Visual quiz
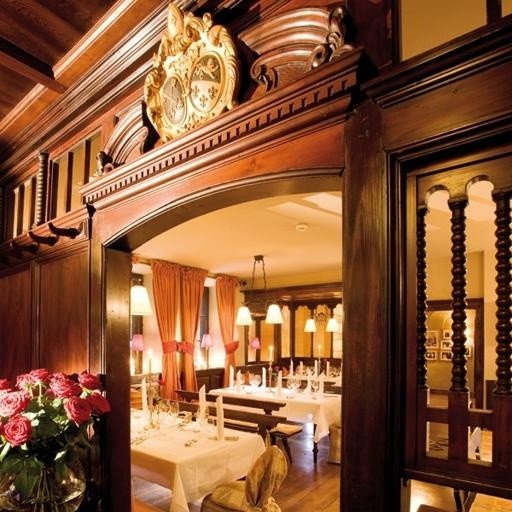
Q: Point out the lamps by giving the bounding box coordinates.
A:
[235,255,283,325]
[325,302,339,333]
[131,334,144,374]
[250,337,261,350]
[130,278,153,339]
[303,303,317,333]
[201,334,214,369]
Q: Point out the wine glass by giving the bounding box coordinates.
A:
[152,399,179,430]
[288,378,301,399]
[235,374,261,395]
[310,380,319,400]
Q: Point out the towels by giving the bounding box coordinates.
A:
[229,359,330,393]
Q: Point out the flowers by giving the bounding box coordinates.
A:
[0,368,112,502]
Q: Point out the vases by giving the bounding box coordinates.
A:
[0,443,87,512]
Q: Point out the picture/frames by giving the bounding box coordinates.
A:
[425,329,471,361]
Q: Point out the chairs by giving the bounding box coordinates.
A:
[416,426,481,512]
